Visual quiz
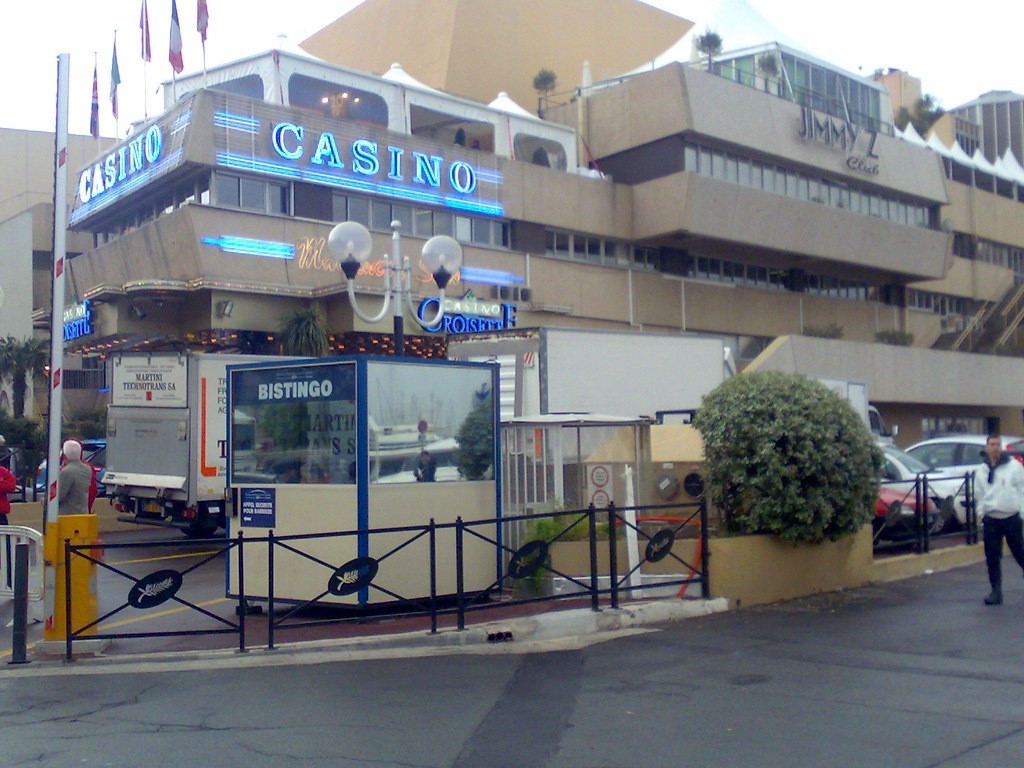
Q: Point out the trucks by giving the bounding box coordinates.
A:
[812,376,897,447]
[450,326,739,426]
[99,350,324,535]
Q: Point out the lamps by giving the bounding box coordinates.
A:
[131,305,147,320]
[221,297,235,318]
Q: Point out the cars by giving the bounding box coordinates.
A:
[7,485,40,503]
[901,435,1024,477]
[875,444,985,536]
[873,489,940,544]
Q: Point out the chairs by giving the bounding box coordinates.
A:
[964,447,982,464]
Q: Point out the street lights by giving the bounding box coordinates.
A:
[327,219,464,356]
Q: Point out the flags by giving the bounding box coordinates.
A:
[88,0,209,140]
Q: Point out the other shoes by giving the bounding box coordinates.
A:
[985,591,1002,604]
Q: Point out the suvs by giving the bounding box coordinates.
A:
[35,437,106,496]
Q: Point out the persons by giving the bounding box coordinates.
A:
[973,433,1024,605]
[0,465,17,589]
[414,451,437,482]
[269,457,373,484]
[58,438,98,514]
